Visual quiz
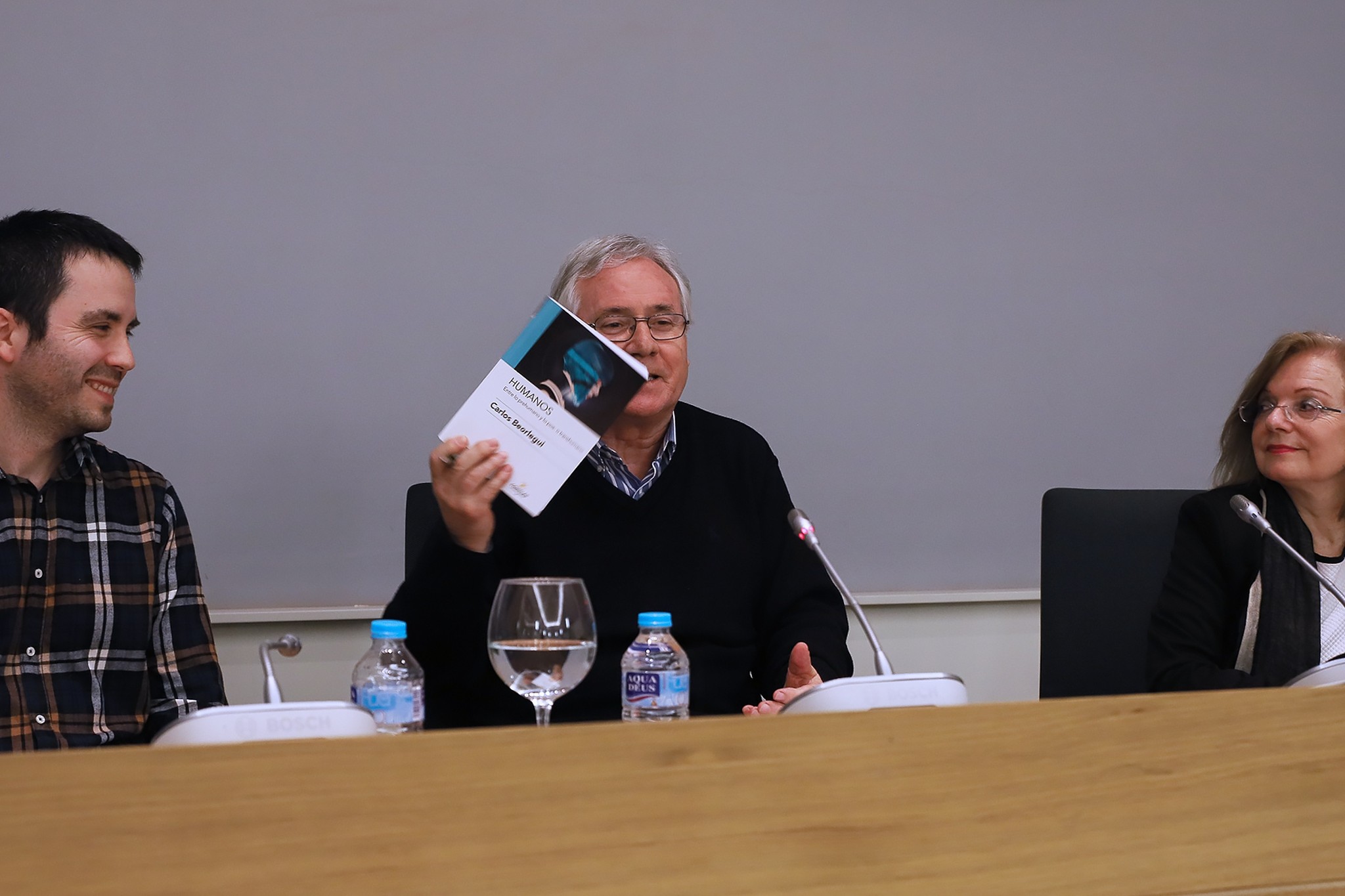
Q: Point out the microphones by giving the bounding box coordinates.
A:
[775,508,969,713]
[1229,494,1345,690]
[150,634,378,746]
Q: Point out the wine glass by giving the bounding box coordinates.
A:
[487,577,596,727]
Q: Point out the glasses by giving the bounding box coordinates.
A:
[589,314,691,343]
[1237,398,1345,423]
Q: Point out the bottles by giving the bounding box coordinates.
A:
[622,610,690,721]
[351,618,425,734]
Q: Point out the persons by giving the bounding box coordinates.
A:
[363,233,851,733]
[1146,330,1345,689]
[0,209,225,751]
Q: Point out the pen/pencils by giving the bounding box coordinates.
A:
[438,455,455,468]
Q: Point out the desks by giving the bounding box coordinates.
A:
[1,684,1345,896]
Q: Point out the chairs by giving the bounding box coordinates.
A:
[1037,488,1208,699]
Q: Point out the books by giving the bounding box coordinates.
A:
[439,297,650,519]
